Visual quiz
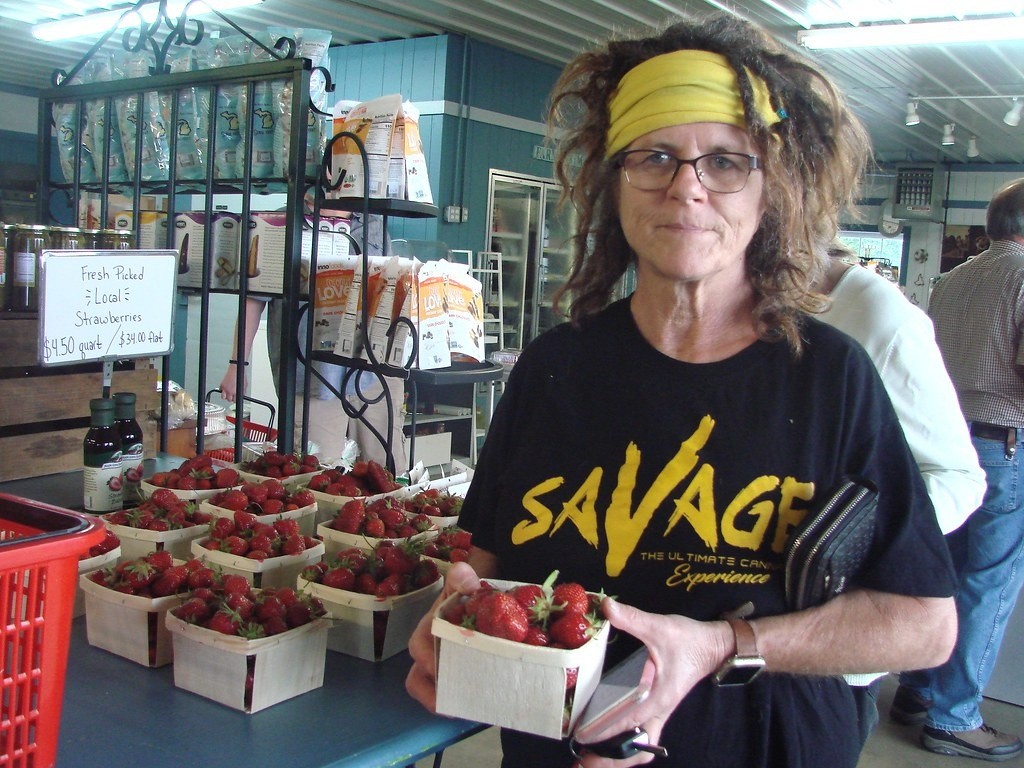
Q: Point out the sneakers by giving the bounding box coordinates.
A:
[889,687,927,725]
[920,724,1022,762]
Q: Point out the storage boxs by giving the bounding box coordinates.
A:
[76,462,462,714]
[404,431,452,470]
[431,578,609,741]
[201,415,278,463]
[80,201,351,295]
[0,491,107,768]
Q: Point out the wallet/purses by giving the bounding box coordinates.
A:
[785,478,880,614]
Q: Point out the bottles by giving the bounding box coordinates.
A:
[112,391,144,510]
[82,397,124,517]
[0,220,138,312]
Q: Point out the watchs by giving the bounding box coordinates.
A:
[710,599,767,689]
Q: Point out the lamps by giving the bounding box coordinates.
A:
[941,123,955,145]
[905,101,920,126]
[966,134,979,157]
[1002,98,1024,127]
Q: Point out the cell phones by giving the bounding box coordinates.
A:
[575,644,657,744]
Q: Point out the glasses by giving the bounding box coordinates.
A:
[617,150,766,193]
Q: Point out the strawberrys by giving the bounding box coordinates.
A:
[80,452,473,696]
[442,570,605,731]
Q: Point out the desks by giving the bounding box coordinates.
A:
[1,454,495,768]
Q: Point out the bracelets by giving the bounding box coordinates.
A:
[229,360,248,366]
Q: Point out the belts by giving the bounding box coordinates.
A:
[970,421,1017,443]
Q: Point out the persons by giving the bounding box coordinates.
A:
[407,10,989,768]
[220,139,406,477]
[890,179,1023,761]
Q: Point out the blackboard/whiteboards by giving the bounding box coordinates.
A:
[38,249,180,368]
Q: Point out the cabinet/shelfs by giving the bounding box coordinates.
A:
[294,131,504,485]
[445,247,504,353]
[402,381,478,477]
[485,175,577,351]
[891,163,945,223]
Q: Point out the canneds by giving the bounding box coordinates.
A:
[0,223,136,312]
